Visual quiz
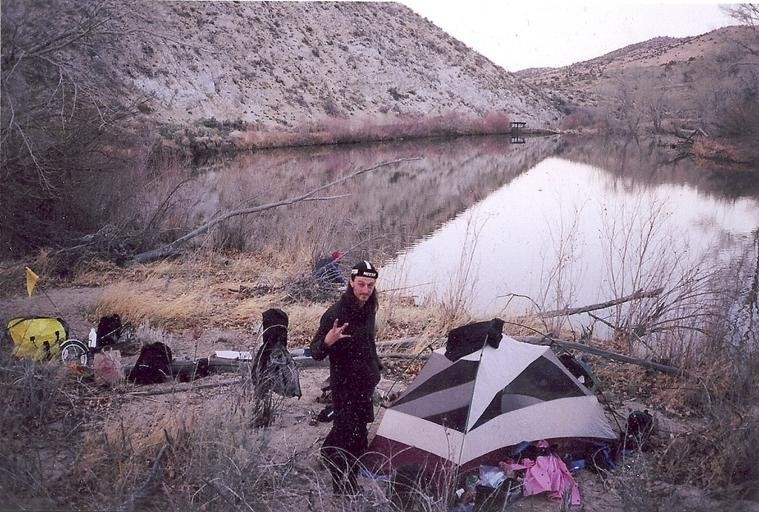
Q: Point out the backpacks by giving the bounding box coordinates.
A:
[619,408,658,452]
[128,341,173,383]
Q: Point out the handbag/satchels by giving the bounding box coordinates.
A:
[5,316,68,362]
[93,345,124,385]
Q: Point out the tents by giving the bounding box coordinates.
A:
[365,330,619,512]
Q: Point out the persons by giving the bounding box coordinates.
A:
[310,260,384,500]
[314,250,348,283]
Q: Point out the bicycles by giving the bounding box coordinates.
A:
[58,321,141,369]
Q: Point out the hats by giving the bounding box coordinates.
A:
[351,260,378,279]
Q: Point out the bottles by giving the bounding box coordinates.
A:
[88,328,97,348]
